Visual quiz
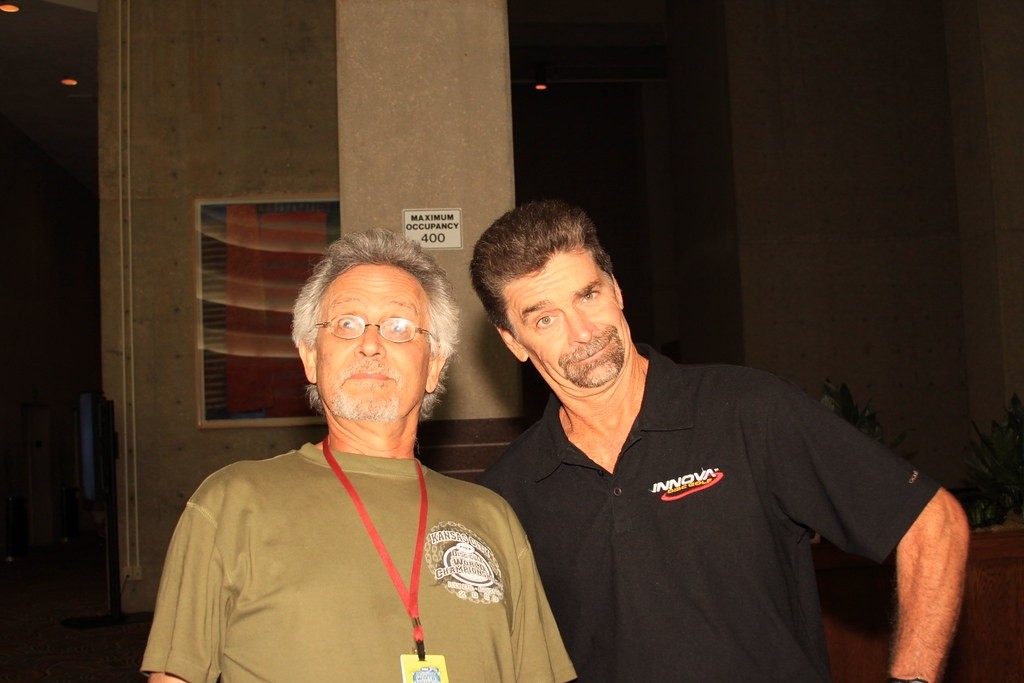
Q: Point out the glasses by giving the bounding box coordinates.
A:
[306,315,439,344]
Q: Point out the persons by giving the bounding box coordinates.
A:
[468,198,970,683]
[137,227,576,683]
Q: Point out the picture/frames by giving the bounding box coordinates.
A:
[193,193,340,428]
